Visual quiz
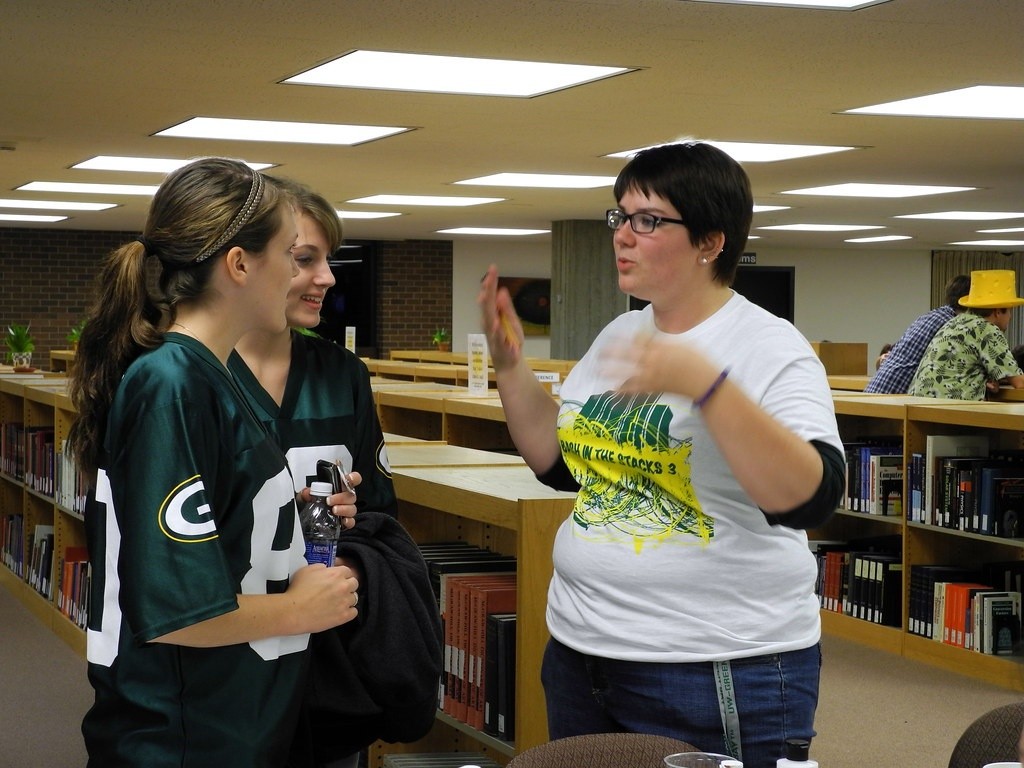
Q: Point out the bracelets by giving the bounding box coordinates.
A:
[691,365,731,409]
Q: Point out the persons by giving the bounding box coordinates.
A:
[65,159,395,768]
[863,270,1024,402]
[479,142,845,766]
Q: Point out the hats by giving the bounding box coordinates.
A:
[958,269,1024,308]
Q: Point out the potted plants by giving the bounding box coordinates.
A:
[4,320,35,368]
[65,318,86,351]
[432,327,451,351]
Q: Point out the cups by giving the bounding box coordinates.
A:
[662,751,743,768]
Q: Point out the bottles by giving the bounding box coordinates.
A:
[298,482,341,568]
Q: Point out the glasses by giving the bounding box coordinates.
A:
[606,208,712,234]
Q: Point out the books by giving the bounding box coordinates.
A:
[809,435,1024,545]
[0,513,93,631]
[405,535,516,747]
[0,423,88,518]
[809,535,1024,657]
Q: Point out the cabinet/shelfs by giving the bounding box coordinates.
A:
[48,350,1024,695]
[348,350,1024,412]
[0,367,580,768]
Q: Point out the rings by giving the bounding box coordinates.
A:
[636,346,651,369]
[352,592,358,607]
